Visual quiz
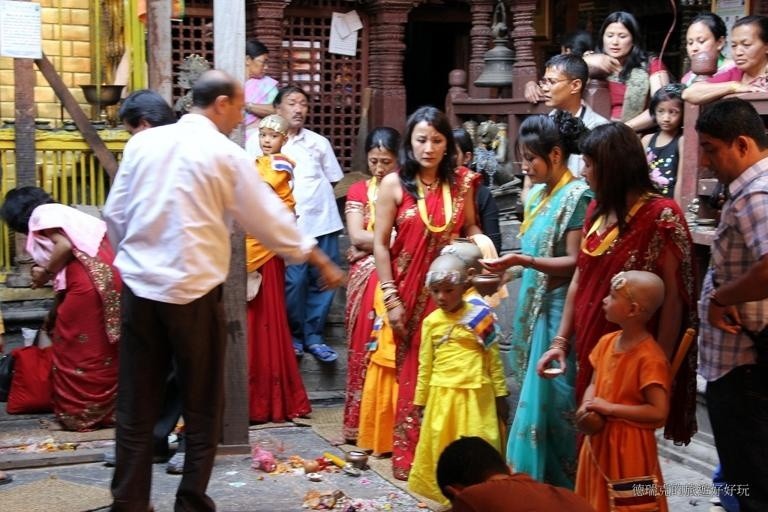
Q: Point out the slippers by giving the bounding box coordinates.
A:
[292,342,304,355]
[308,346,339,362]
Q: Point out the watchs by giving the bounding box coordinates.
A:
[44,268,55,277]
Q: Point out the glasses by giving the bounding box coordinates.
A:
[610,268,632,299]
[539,78,572,88]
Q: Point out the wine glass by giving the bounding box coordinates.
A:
[79,81,124,127]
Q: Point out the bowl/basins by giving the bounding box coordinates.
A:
[471,273,501,298]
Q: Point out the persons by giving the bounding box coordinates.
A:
[249,6,768,512]
[100,68,352,512]
[104,88,190,474]
[0,185,125,428]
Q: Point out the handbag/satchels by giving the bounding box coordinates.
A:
[608,476,660,510]
[7,348,53,414]
